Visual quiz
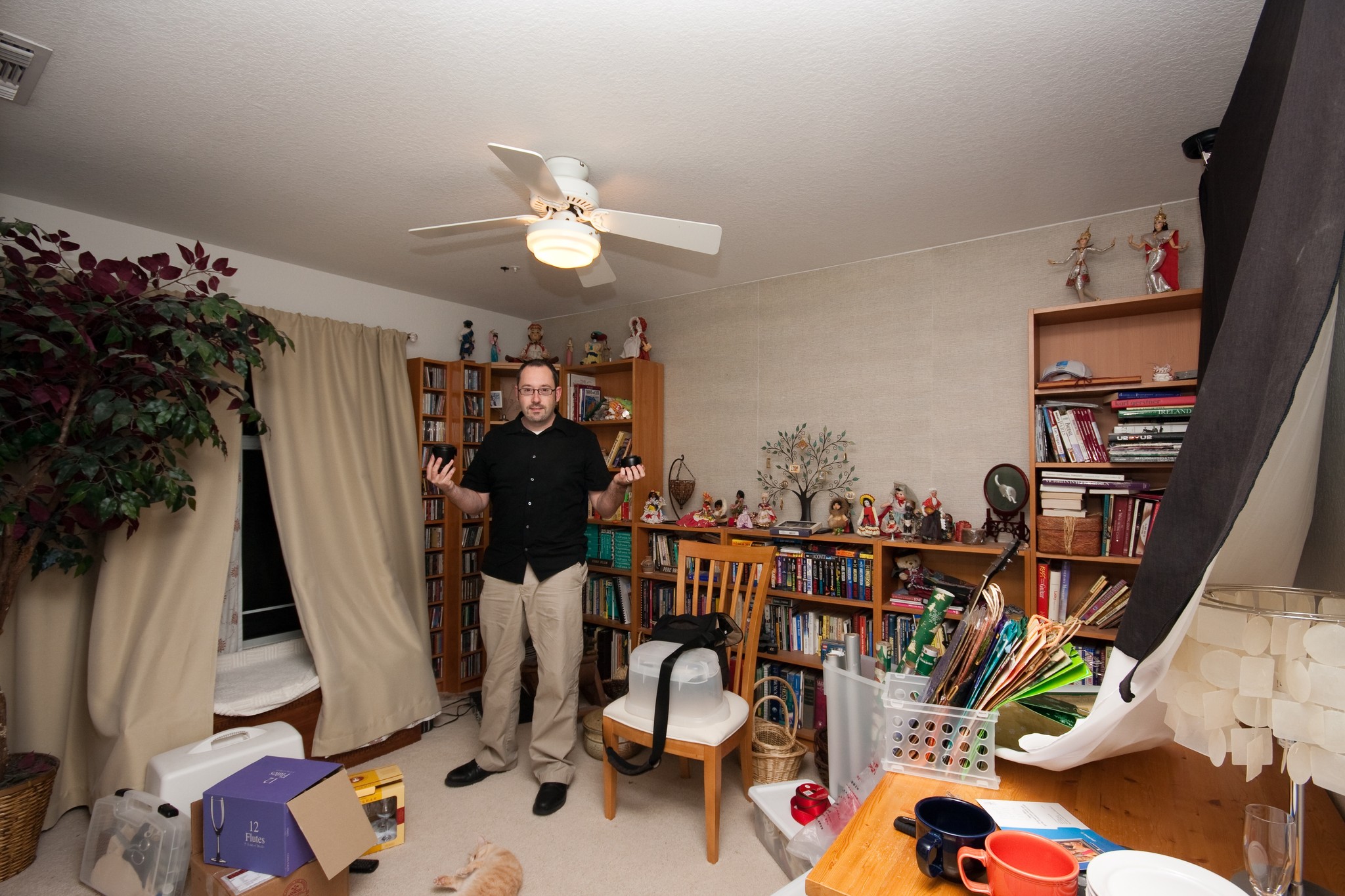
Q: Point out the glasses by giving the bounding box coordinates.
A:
[518,388,557,395]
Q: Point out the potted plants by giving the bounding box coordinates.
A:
[0,216,295,881]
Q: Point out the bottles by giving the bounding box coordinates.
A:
[642,556,654,574]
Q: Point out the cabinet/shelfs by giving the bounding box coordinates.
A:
[402,288,1201,755]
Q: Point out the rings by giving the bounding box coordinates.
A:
[626,478,630,484]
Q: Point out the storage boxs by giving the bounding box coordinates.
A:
[79,721,406,896]
[748,779,836,881]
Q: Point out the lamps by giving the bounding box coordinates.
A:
[527,219,601,269]
[1154,580,1345,896]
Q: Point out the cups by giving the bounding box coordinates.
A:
[957,830,1080,896]
[1242,804,1296,896]
[914,796,996,884]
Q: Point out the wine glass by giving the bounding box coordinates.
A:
[210,796,226,864]
[373,796,397,845]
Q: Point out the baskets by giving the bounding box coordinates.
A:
[730,677,808,785]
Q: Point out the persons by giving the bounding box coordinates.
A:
[827,497,851,536]
[641,489,777,529]
[880,481,944,544]
[793,466,825,479]
[426,358,645,816]
[1047,201,1190,303]
[856,494,880,537]
[457,316,654,363]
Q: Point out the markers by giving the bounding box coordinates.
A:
[893,816,916,838]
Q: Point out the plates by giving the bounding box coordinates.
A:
[1085,850,1250,896]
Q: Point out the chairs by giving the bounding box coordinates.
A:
[602,539,777,865]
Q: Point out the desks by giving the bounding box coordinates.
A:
[805,748,1345,896]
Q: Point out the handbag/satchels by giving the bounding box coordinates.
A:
[652,610,744,681]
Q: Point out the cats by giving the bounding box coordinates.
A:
[432,835,523,896]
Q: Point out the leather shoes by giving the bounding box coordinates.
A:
[444,758,496,788]
[532,781,568,816]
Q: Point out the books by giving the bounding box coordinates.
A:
[640,532,722,629]
[566,372,631,569]
[882,614,957,665]
[422,364,482,679]
[1034,389,1196,555]
[584,628,631,680]
[884,561,1132,777]
[730,539,872,729]
[584,576,630,625]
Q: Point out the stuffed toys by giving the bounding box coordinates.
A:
[895,555,934,598]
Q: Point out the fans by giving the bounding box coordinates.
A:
[408,143,722,288]
